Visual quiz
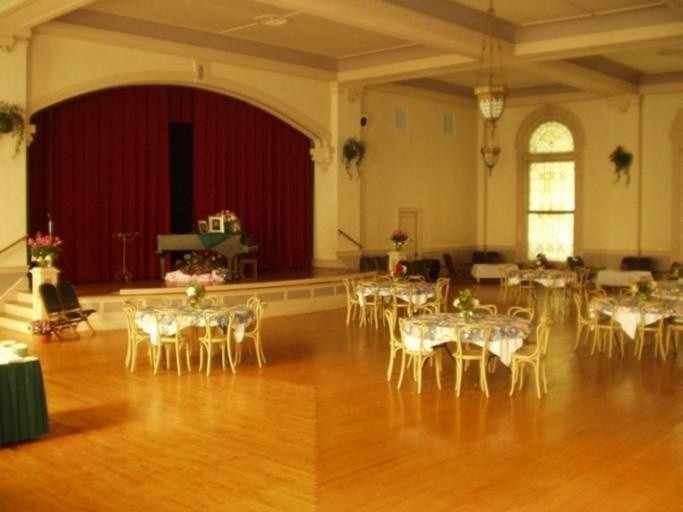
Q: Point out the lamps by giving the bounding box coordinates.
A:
[473,0,506,179]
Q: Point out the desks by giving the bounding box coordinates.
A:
[0,340,49,444]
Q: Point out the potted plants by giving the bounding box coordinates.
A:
[342,138,365,179]
[0,100,25,160]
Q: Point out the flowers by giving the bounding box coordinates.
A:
[28,231,62,267]
[216,209,241,233]
[388,228,409,251]
[176,250,222,276]
[184,281,205,307]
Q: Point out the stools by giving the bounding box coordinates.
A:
[239,259,259,282]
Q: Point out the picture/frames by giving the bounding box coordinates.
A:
[208,215,224,234]
[197,220,208,234]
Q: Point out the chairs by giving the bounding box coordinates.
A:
[56,283,98,339]
[123,294,266,375]
[37,282,81,341]
